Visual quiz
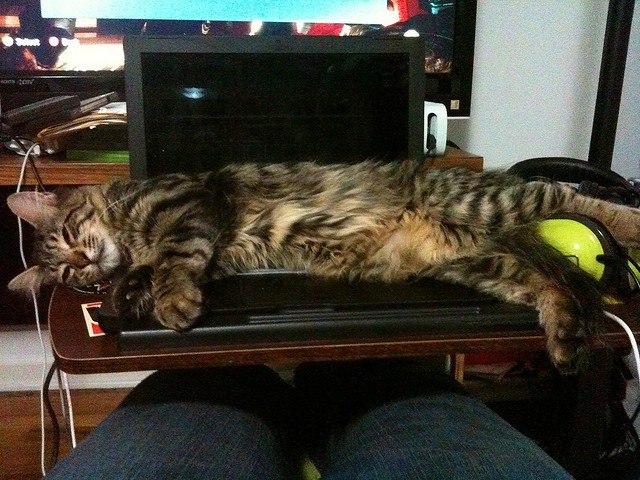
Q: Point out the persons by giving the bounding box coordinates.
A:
[39,361,577,480]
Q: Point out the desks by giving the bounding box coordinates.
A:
[47,272,640,382]
[0,132,483,187]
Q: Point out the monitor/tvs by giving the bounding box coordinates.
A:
[1,1,477,122]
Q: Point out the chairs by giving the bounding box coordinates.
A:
[506,157,631,196]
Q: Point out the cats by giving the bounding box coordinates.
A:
[7,152,640,378]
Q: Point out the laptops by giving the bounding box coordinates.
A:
[118,32,541,344]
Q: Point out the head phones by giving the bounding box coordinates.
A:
[506,157,640,294]
